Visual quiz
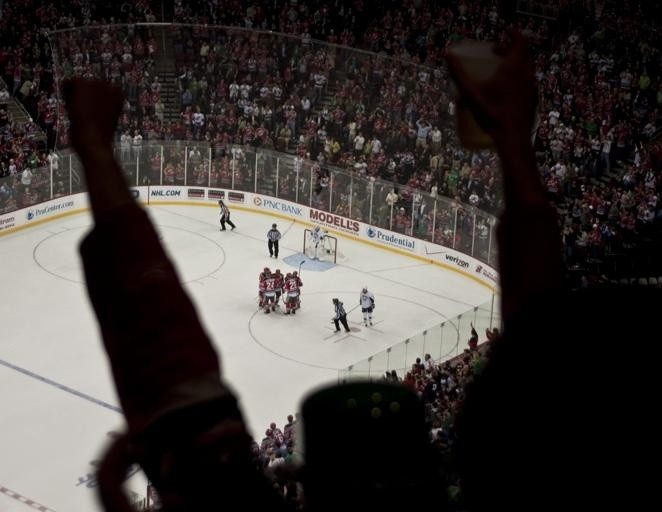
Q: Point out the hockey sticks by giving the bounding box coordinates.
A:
[331,304,360,324]
[282,292,286,303]
[299,259,305,277]
[279,218,295,240]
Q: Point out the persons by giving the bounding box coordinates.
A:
[61,44,580,512]
[251,268,661,511]
[1,1,661,271]
[93,424,158,510]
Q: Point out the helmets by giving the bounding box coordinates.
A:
[363,289,366,293]
[218,200,221,203]
[264,268,297,279]
[333,299,337,303]
[272,225,277,227]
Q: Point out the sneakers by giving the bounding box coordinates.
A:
[365,322,373,326]
[259,301,296,314]
[220,228,226,231]
[334,328,350,334]
[231,228,234,230]
[270,253,278,259]
